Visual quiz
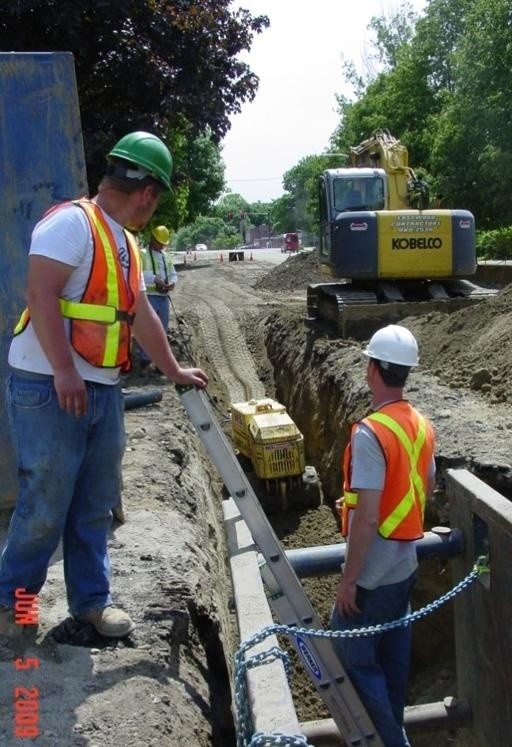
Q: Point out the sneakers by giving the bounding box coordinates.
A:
[75,605,137,638]
[1,609,38,639]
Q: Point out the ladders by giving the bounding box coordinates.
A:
[172,381,384,747]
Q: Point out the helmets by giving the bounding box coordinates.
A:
[362,324,420,367]
[151,225,171,246]
[106,130,176,199]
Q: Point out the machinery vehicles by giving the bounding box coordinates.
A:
[304,128,502,342]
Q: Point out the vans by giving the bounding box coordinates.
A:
[281,233,299,253]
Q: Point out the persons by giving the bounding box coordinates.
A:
[330,323,438,746]
[2,129,210,640]
[140,225,177,378]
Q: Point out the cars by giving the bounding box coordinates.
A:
[195,244,207,251]
